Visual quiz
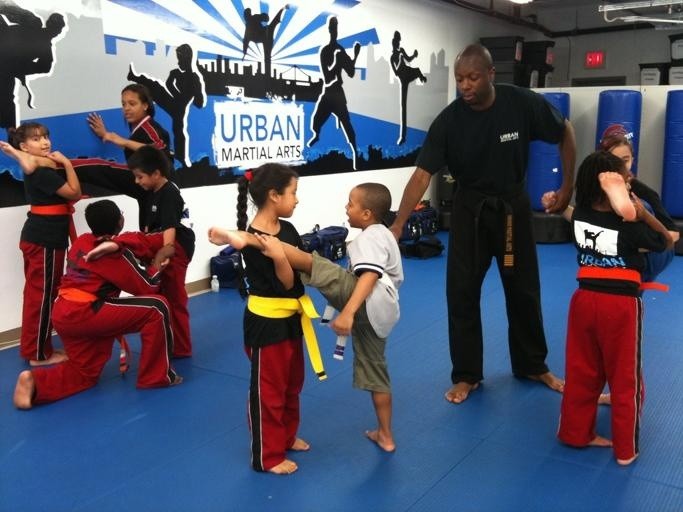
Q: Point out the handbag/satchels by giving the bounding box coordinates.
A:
[208,245,241,289]
[299,233,320,256]
[316,225,349,260]
[384,207,439,240]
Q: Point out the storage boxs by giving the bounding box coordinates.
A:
[478,35,555,88]
[638,33,683,85]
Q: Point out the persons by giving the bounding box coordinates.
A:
[515,13,558,88]
[82,143,197,358]
[8,119,82,366]
[240,3,291,76]
[389,42,576,403]
[305,15,364,170]
[210,180,405,453]
[0,84,177,281]
[126,43,208,169]
[13,200,186,413]
[234,160,313,476]
[387,31,429,148]
[557,148,675,465]
[541,121,681,407]
[0,1,66,146]
